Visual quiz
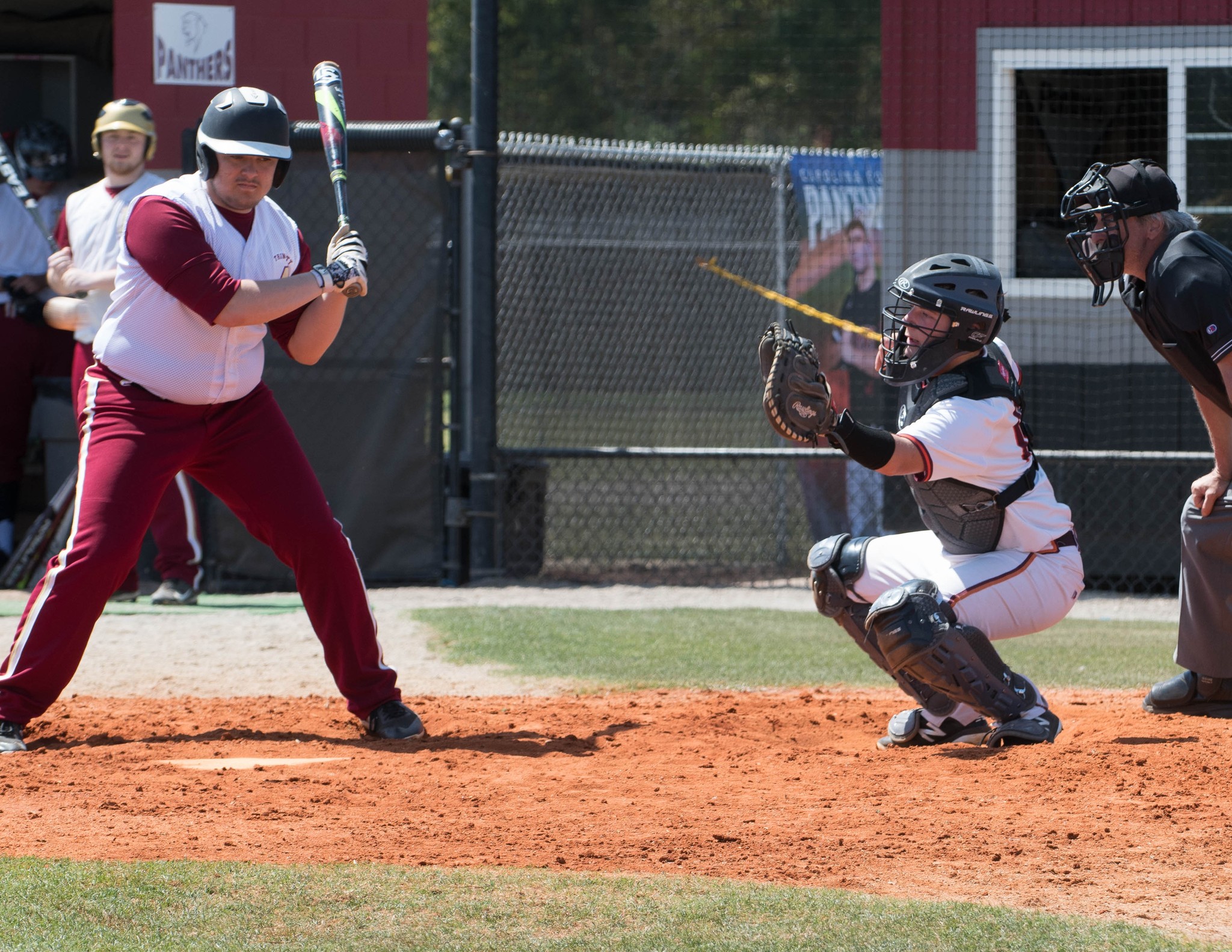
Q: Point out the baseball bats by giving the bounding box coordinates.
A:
[0,141,89,302]
[311,60,362,299]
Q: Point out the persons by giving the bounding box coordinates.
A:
[825,219,884,538]
[0,116,76,546]
[1061,157,1232,714]
[46,98,205,606]
[0,83,428,754]
[759,252,1084,748]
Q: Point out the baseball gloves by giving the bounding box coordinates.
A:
[757,319,828,448]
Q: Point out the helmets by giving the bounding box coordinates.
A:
[878,251,1012,386]
[196,87,294,190]
[12,118,76,181]
[91,97,158,162]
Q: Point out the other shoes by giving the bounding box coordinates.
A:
[1141,667,1232,714]
[0,518,16,556]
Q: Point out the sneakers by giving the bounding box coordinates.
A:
[875,701,994,751]
[149,578,198,605]
[0,719,28,754]
[1000,672,1062,746]
[107,588,142,603]
[358,697,425,741]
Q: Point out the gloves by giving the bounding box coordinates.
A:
[311,222,370,297]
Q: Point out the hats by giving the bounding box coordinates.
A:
[1081,162,1180,212]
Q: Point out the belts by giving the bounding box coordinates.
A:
[102,367,166,401]
